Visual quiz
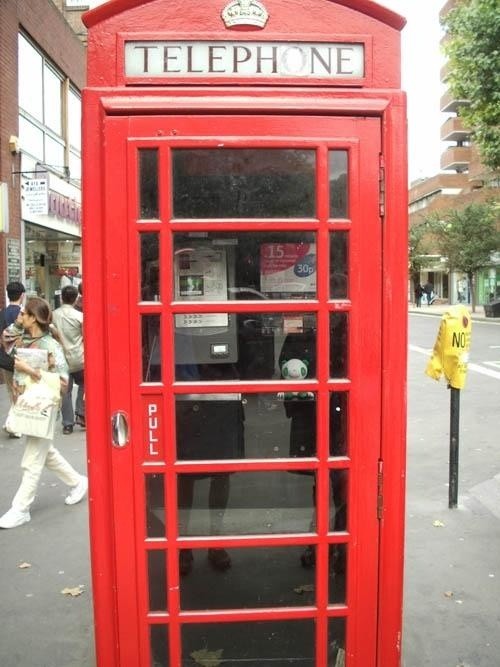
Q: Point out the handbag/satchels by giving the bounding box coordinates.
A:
[6,398,58,440]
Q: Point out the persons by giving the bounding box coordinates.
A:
[48,285,85,435]
[147,313,246,576]
[0,281,29,439]
[423,280,434,305]
[74,282,84,311]
[278,273,348,575]
[414,283,425,307]
[1,295,89,528]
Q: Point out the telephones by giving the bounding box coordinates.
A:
[144,237,238,367]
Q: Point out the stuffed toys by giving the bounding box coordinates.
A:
[276,358,315,400]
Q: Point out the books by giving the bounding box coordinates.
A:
[15,347,48,385]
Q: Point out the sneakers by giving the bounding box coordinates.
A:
[180,550,194,573]
[63,425,73,434]
[0,507,31,529]
[10,432,21,438]
[301,543,346,573]
[208,548,231,570]
[76,415,84,427]
[65,476,88,505]
